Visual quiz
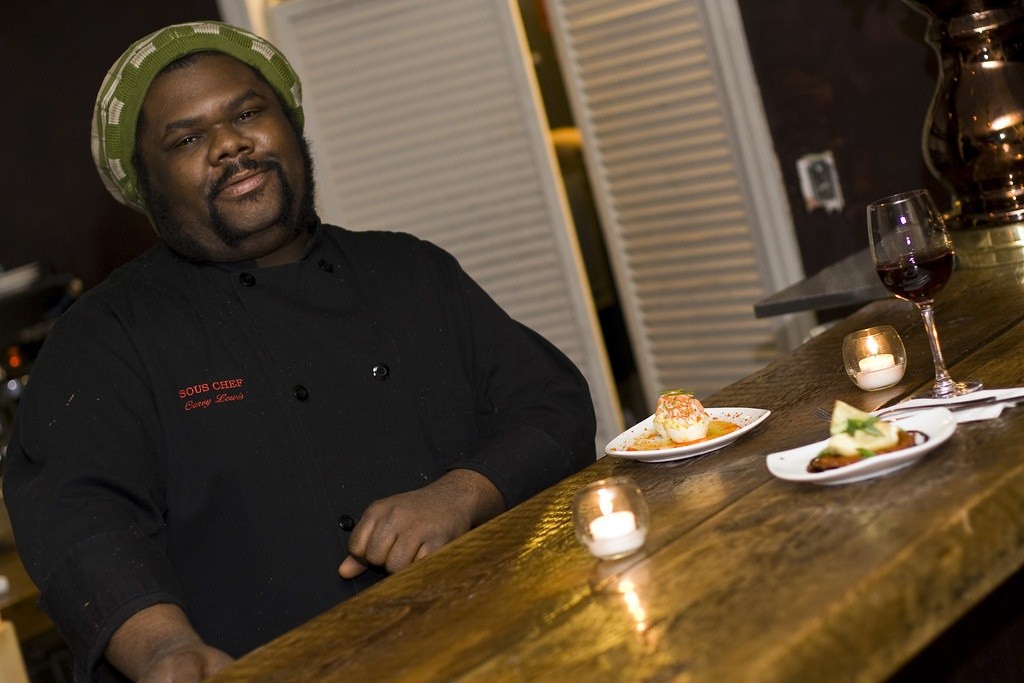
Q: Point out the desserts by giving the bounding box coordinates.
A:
[654,388,710,443]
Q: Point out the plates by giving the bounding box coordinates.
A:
[766,406,957,486]
[604,406,771,462]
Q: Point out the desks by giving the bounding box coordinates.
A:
[204,262,1024,683]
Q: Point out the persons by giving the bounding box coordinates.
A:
[3,21,596,683]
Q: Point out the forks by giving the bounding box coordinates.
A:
[812,396,996,424]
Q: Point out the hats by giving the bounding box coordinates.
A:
[92,19,304,235]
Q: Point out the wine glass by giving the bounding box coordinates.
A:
[867,188,982,398]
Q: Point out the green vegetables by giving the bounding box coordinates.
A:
[844,418,884,459]
